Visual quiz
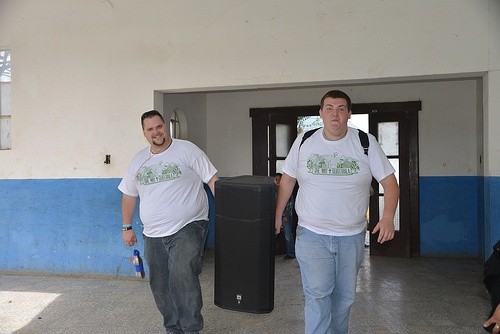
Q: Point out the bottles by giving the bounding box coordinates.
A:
[133,249,145,280]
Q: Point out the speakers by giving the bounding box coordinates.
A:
[212,175,278,314]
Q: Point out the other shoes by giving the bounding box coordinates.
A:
[283,255,294,259]
